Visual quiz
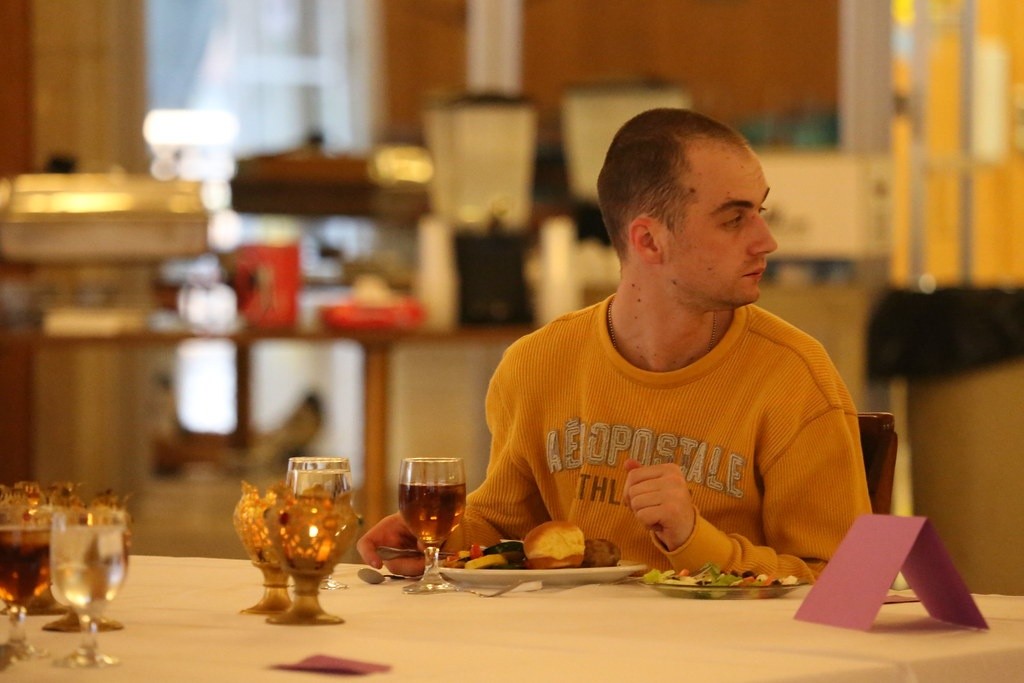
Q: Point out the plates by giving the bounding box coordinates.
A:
[320,305,424,335]
[637,580,809,599]
[436,559,648,588]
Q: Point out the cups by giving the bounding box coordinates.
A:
[234,240,299,330]
[293,469,351,499]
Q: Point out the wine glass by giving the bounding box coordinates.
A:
[49,510,128,668]
[0,514,52,662]
[284,457,357,589]
[397,458,466,594]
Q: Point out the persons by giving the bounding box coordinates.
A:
[354,107,872,587]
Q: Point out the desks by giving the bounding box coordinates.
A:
[0,552,1024,683]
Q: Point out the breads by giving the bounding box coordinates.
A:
[524,520,585,567]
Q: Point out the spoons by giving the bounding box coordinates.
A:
[377,540,524,568]
[358,568,406,584]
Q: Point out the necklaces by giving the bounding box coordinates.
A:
[608,293,717,355]
